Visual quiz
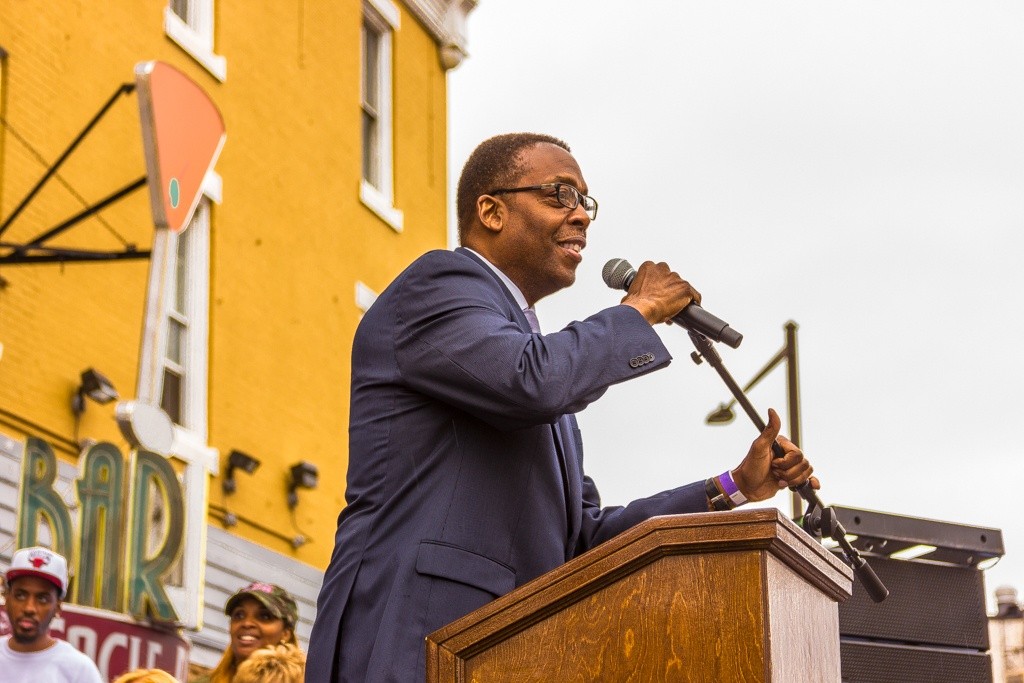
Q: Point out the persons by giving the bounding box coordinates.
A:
[304,139,822,682]
[0,546,306,683]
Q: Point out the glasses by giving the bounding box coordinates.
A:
[489,183,597,220]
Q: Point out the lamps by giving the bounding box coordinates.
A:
[72,369,118,413]
[224,450,259,493]
[289,462,318,507]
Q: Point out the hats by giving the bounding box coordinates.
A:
[225,583,297,629]
[5,546,67,601]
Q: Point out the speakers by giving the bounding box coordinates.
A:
[826,545,994,683]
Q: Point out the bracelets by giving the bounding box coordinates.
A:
[720,469,750,507]
[705,477,731,512]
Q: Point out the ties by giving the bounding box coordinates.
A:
[523,310,540,334]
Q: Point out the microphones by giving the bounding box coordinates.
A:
[601,259,743,349]
